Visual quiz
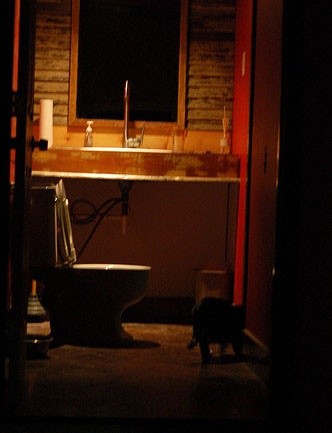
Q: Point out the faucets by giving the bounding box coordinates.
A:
[122,79,132,148]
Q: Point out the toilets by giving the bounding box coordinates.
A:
[28,178,151,342]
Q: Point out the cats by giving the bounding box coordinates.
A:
[186,295,247,364]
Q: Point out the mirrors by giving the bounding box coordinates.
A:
[68,1,188,134]
